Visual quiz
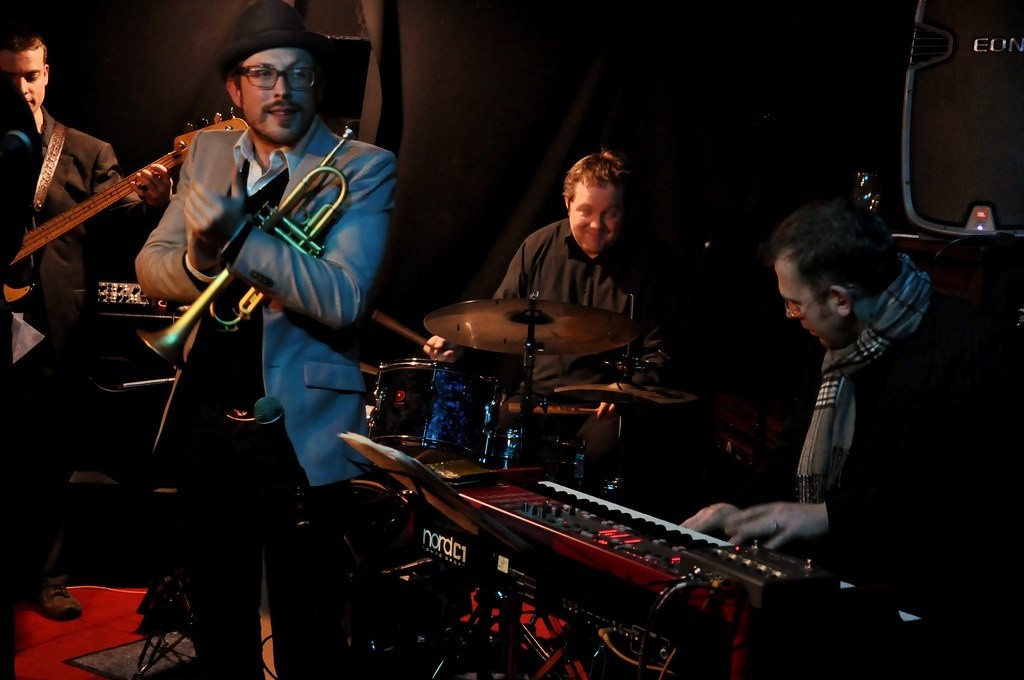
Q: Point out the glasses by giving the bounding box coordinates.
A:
[229,64,322,92]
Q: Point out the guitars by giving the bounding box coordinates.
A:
[0,114,257,301]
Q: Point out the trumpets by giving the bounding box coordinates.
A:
[139,125,361,372]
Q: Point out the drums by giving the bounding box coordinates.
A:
[364,357,505,465]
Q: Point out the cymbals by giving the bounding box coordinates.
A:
[551,382,702,407]
[421,295,638,355]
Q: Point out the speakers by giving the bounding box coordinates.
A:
[902,0,1024,239]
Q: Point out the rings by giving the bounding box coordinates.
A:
[772,520,779,529]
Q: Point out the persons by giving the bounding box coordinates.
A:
[0,30,173,620]
[135,0,397,680]
[424,146,673,495]
[680,197,1024,680]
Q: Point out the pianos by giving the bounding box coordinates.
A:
[385,456,927,646]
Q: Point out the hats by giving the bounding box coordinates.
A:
[214,0,334,87]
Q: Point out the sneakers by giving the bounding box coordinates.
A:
[33,584,82,621]
